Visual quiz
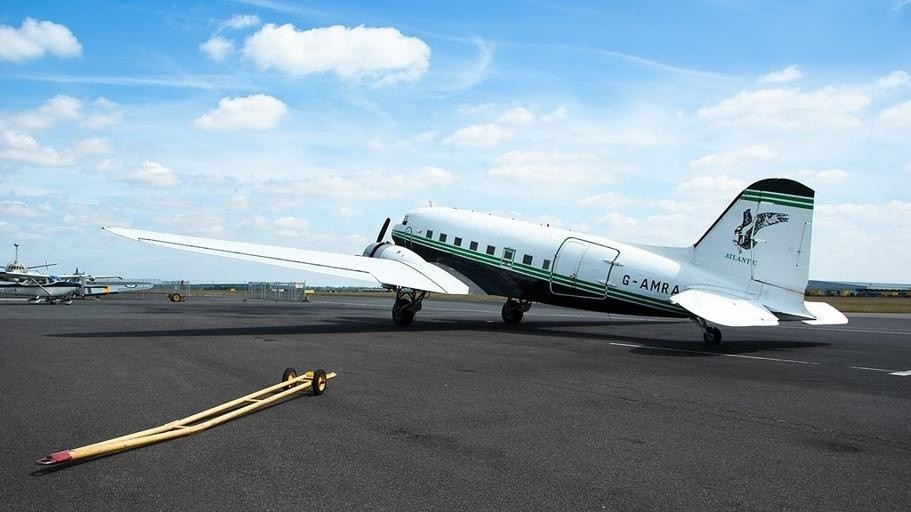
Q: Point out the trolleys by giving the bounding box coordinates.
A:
[34,367,341,468]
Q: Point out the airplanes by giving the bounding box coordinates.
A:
[0,243,154,305]
[98,176,851,346]
